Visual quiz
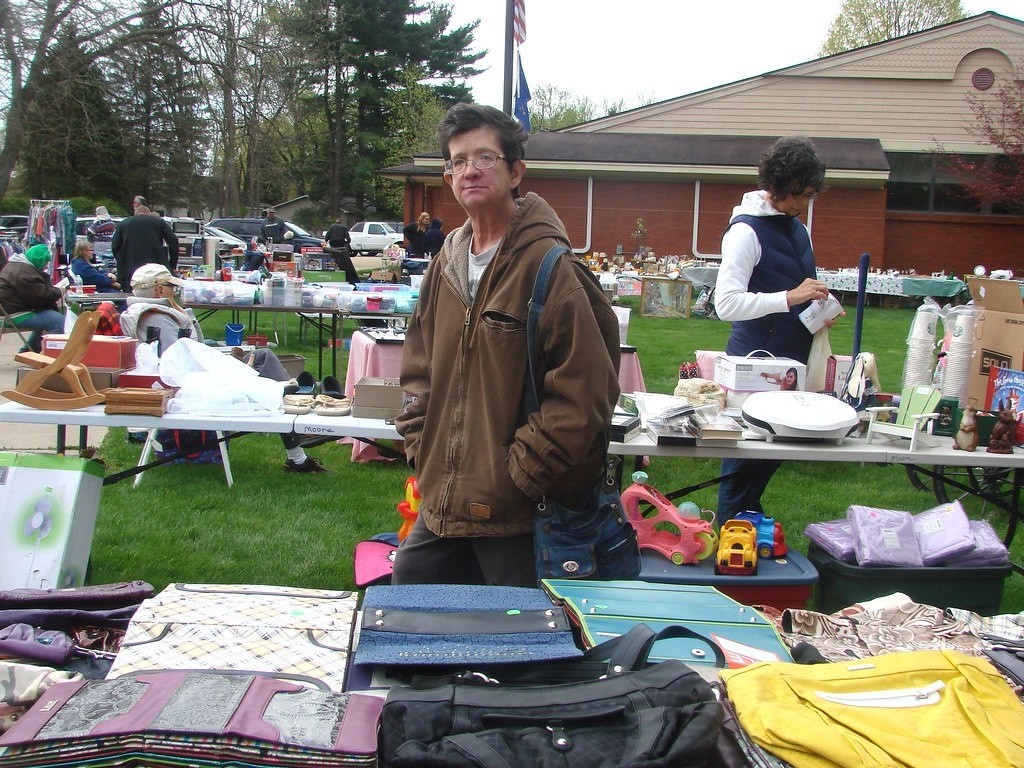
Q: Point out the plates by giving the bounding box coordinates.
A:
[974,265,985,275]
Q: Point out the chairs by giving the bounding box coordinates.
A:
[0,311,115,410]
[0,304,34,352]
[865,385,942,452]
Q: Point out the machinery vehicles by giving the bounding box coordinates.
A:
[735,510,788,560]
[714,519,757,576]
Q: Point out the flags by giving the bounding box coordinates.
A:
[514,50,532,132]
[514,0,527,47]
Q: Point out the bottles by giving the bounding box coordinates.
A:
[75,275,83,295]
[586,252,614,271]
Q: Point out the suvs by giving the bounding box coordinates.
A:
[210,216,329,254]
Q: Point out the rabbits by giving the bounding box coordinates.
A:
[952,400,979,452]
[986,397,1014,455]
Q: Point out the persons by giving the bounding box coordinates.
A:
[714,136,846,536]
[0,244,66,354]
[390,102,622,586]
[402,212,447,258]
[86,206,116,257]
[119,263,330,476]
[775,368,799,391]
[260,207,286,245]
[111,195,179,293]
[70,241,128,314]
[325,218,351,248]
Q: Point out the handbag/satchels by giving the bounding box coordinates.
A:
[717,650,1024,768]
[381,624,783,768]
[157,430,231,466]
[534,475,643,590]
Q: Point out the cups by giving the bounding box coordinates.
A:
[903,312,937,389]
[943,315,973,409]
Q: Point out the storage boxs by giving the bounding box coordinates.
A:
[807,541,1013,617]
[639,545,818,610]
[181,270,425,314]
[265,245,336,278]
[277,355,306,378]
[0,449,106,591]
[714,350,807,407]
[15,334,180,393]
[825,354,852,398]
[246,335,268,346]
[352,376,407,419]
[966,277,1024,411]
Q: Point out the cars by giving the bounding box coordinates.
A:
[0,215,28,243]
[74,215,127,243]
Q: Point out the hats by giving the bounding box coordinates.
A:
[267,207,276,212]
[130,262,186,289]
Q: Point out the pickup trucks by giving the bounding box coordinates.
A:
[322,222,405,257]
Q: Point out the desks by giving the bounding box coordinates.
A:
[336,331,650,466]
[607,394,1024,519]
[381,258,430,283]
[57,264,117,276]
[181,298,412,382]
[681,267,966,309]
[0,400,404,485]
[66,293,135,314]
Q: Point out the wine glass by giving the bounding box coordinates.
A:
[870,266,873,273]
[902,269,908,275]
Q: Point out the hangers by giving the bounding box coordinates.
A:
[31,199,73,211]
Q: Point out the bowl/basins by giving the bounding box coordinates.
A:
[409,275,424,290]
[69,285,96,293]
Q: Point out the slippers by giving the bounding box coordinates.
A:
[280,393,351,416]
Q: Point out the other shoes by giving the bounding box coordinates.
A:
[298,434,346,449]
[283,454,336,474]
[295,371,346,398]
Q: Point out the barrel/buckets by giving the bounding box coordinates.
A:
[329,338,351,349]
[225,324,245,346]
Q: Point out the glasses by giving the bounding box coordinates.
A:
[788,191,819,200]
[161,284,178,293]
[443,151,507,175]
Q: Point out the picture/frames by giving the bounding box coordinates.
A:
[640,277,692,319]
